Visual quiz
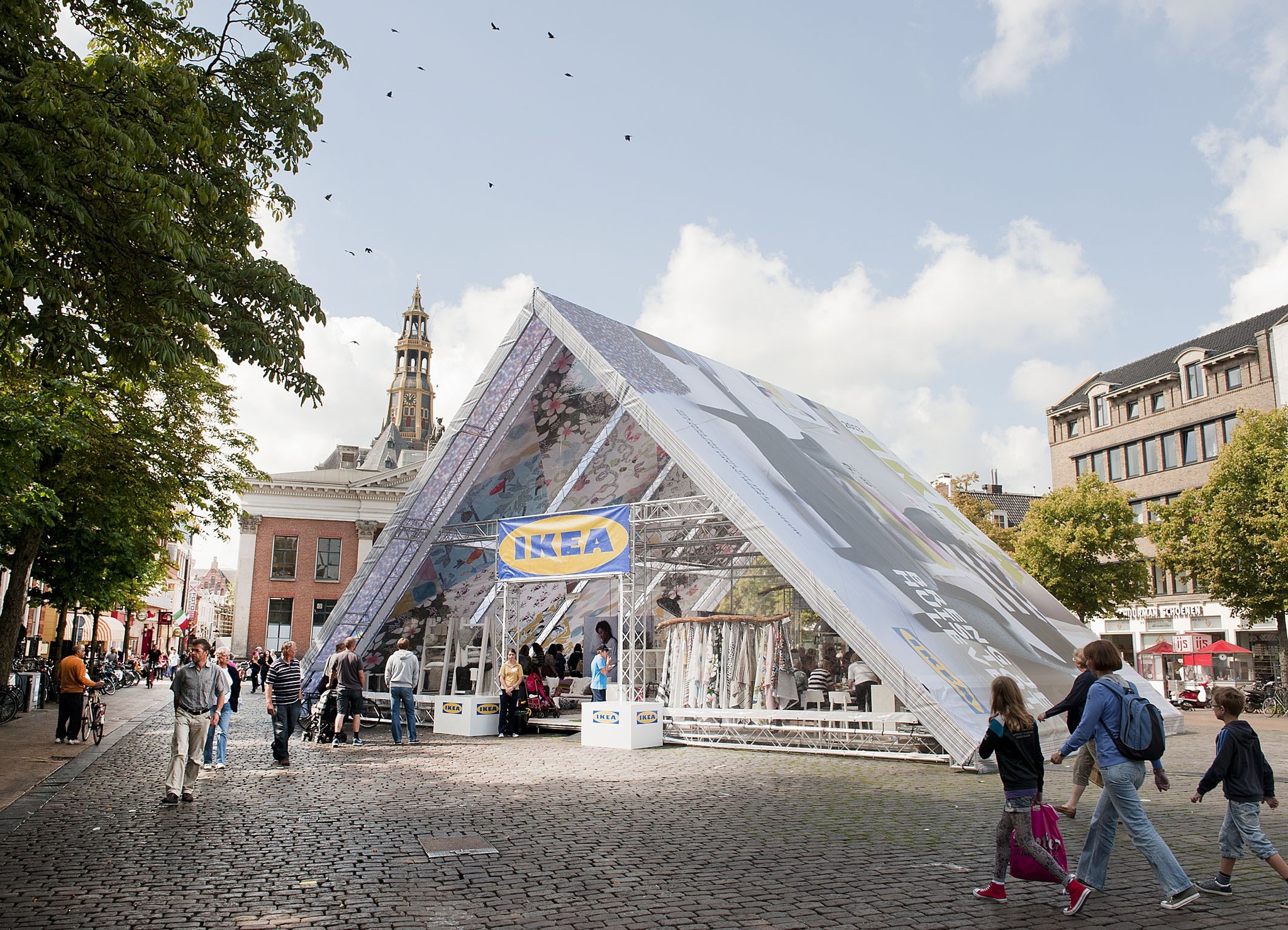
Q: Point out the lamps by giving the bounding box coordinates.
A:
[152,626,154,631]
[141,626,147,635]
[657,566,682,618]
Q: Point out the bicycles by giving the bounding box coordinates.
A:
[1261,687,1288,718]
[80,682,107,744]
[349,696,384,728]
[0,654,167,724]
[236,660,253,682]
[298,692,322,730]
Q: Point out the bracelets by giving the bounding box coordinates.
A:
[1153,769,1165,774]
[215,711,221,716]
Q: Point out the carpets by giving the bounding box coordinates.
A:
[549,709,581,716]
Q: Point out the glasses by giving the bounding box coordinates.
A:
[1209,703,1227,711]
[1072,658,1077,663]
[191,648,204,654]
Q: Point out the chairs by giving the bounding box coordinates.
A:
[368,699,434,724]
[827,691,863,740]
[803,691,830,738]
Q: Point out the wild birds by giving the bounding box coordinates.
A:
[390,28,400,34]
[564,72,575,78]
[344,249,356,256]
[348,340,360,345]
[488,182,495,188]
[259,249,268,257]
[365,247,373,254]
[547,31,556,39]
[490,21,500,31]
[623,135,633,142]
[436,384,439,387]
[305,161,312,166]
[416,65,426,71]
[323,193,332,202]
[319,138,328,144]
[386,90,394,98]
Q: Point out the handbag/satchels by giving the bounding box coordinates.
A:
[257,673,262,685]
[317,674,338,694]
[1009,801,1069,884]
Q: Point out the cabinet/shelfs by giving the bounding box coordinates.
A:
[417,618,454,696]
[451,616,490,696]
[622,649,665,686]
[362,674,385,704]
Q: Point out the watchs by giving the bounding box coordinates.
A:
[513,686,515,689]
[1058,749,1065,757]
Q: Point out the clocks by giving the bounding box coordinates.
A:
[403,393,416,405]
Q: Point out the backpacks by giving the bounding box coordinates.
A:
[1094,679,1166,761]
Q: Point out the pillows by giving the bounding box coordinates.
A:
[558,679,573,693]
[426,655,455,670]
[467,651,492,663]
[580,678,593,696]
[559,698,579,710]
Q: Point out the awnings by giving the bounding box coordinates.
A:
[55,613,93,641]
[97,615,125,640]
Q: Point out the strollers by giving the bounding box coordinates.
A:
[302,686,348,743]
[528,672,561,719]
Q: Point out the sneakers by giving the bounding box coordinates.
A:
[1063,878,1092,916]
[1279,898,1288,908]
[1195,878,1233,896]
[332,738,340,747]
[203,762,225,769]
[973,881,1007,903]
[1069,874,1097,892]
[352,738,363,745]
[1160,886,1200,909]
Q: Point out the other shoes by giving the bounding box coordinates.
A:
[508,733,519,737]
[250,689,265,694]
[170,676,173,681]
[498,733,504,737]
[56,737,80,744]
[1052,804,1076,819]
[396,741,402,745]
[146,683,148,686]
[408,739,421,744]
[271,744,290,766]
[165,792,193,803]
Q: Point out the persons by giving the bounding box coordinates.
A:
[1179,664,1191,681]
[596,620,618,683]
[1038,646,1123,824]
[1190,687,1288,907]
[974,677,1093,916]
[1143,664,1151,679]
[770,645,879,734]
[383,638,421,745]
[107,637,365,803]
[55,644,105,744]
[1051,641,1201,909]
[498,642,614,738]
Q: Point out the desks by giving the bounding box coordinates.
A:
[871,685,904,731]
[433,695,501,737]
[581,701,663,750]
[362,691,435,703]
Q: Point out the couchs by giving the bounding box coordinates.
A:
[542,676,611,709]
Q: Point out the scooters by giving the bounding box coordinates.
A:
[1177,680,1214,710]
[1242,676,1281,713]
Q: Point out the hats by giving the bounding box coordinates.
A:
[597,644,609,651]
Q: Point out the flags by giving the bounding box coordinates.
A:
[173,608,189,630]
[174,612,189,628]
